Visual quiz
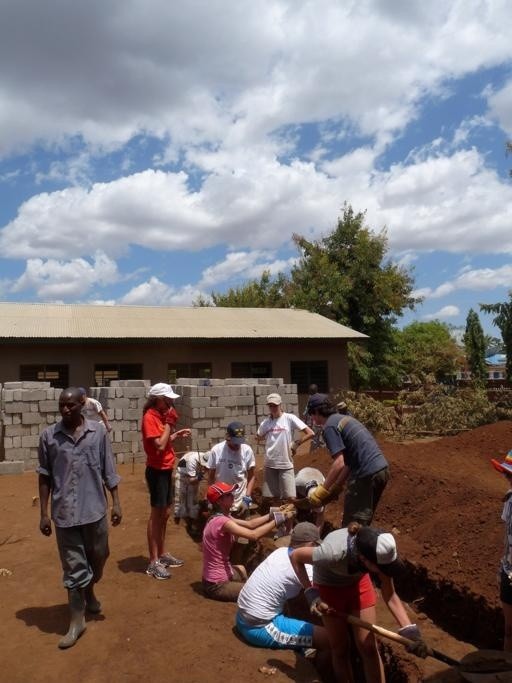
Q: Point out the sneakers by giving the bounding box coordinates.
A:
[145,560,171,580]
[158,552,184,568]
[273,527,286,540]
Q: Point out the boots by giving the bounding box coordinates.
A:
[86,582,101,612]
[59,588,87,648]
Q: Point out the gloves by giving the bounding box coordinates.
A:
[308,484,331,507]
[165,406,179,425]
[270,503,298,525]
[398,623,427,659]
[236,496,252,517]
[304,586,329,617]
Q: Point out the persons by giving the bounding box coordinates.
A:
[175,393,429,683]
[36,385,123,649]
[78,388,112,435]
[491,449,512,671]
[141,383,193,581]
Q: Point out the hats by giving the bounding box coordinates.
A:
[227,422,246,445]
[207,482,239,504]
[148,382,181,399]
[200,451,211,467]
[491,450,512,476]
[337,402,347,409]
[357,528,406,577]
[291,521,323,545]
[303,392,331,416]
[265,393,282,405]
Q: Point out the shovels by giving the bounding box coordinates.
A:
[316,603,512,683]
